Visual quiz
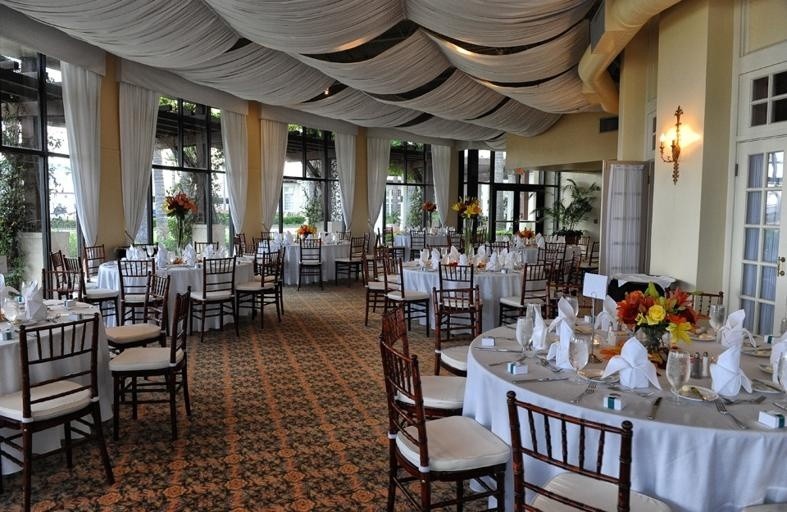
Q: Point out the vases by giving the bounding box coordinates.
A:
[463,218,472,257]
[176,217,184,248]
[427,211,432,226]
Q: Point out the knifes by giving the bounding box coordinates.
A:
[512,377,569,384]
[647,396,662,420]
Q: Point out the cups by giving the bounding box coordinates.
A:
[565,295,580,317]
[526,302,542,328]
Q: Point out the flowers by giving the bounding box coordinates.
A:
[163,186,198,228]
[420,201,437,214]
[450,194,483,220]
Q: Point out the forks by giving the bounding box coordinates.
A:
[572,381,599,403]
[489,356,528,367]
[713,399,747,432]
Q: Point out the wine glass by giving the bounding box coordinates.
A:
[515,316,534,358]
[0,282,91,343]
[400,225,454,235]
[492,233,566,248]
[665,350,692,404]
[416,244,524,273]
[269,230,340,245]
[709,304,727,347]
[567,335,589,385]
[120,243,233,269]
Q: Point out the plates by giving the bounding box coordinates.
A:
[742,346,771,358]
[759,364,774,375]
[670,385,718,403]
[749,377,785,394]
[577,368,619,385]
[689,334,717,342]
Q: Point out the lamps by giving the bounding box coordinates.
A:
[658,105,683,186]
